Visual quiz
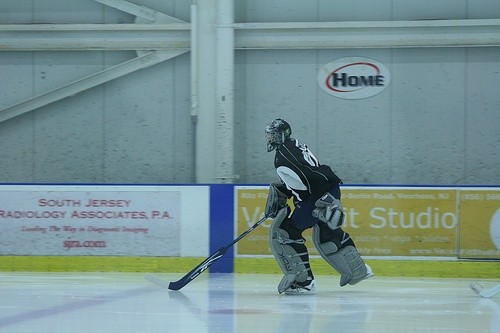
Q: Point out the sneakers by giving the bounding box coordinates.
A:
[285,280,318,295]
[348,262,374,285]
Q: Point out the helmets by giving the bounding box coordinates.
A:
[264,117,292,152]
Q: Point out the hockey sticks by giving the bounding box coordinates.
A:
[168,205,286,292]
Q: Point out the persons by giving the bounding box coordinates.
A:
[265,118,374,295]
